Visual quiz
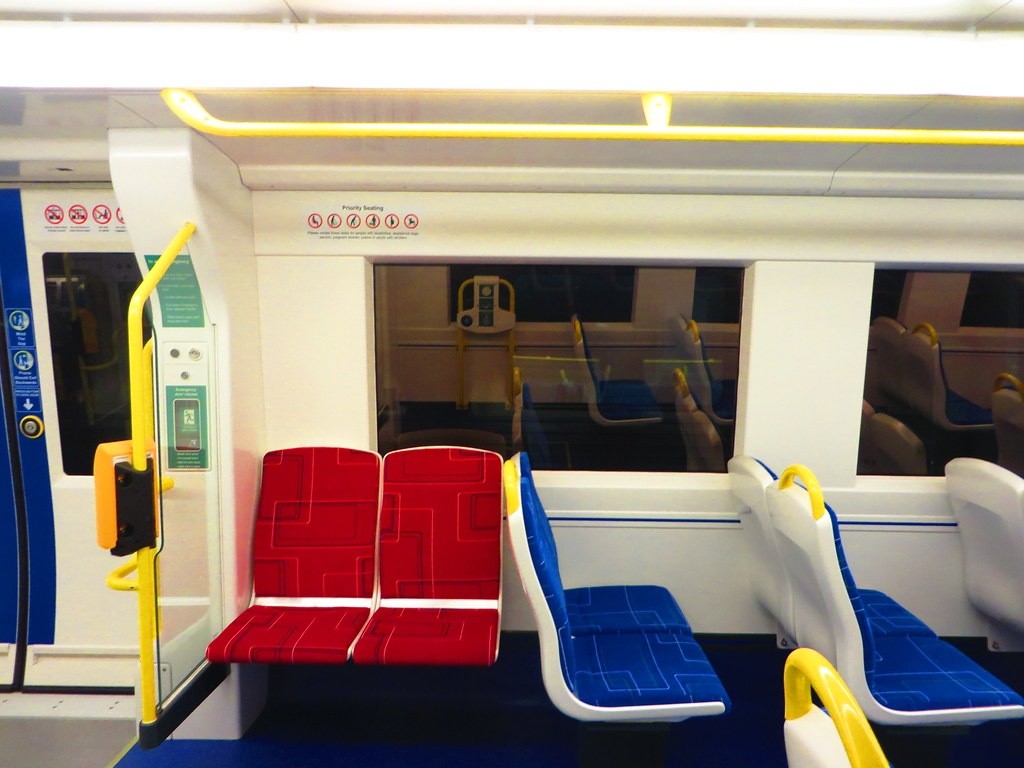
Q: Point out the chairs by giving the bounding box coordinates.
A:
[784,647,895,768]
[511,452,694,636]
[857,318,1024,478]
[944,458,1024,697]
[766,464,1024,768]
[205,446,382,667]
[727,456,937,650]
[502,460,731,767]
[351,445,503,666]
[511,313,737,473]
